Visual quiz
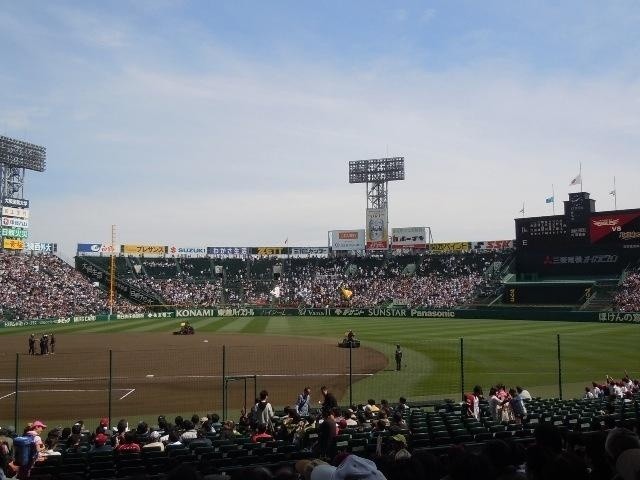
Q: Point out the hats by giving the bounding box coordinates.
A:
[72,423,82,434]
[296,458,327,475]
[310,455,388,480]
[94,433,108,445]
[388,434,407,448]
[151,431,160,438]
[99,416,108,427]
[32,420,47,429]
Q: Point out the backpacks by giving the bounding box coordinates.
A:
[13,434,39,468]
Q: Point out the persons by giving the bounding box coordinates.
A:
[1,384,640,479]
[583,370,639,398]
[180,319,190,335]
[0,248,515,321]
[28,332,56,356]
[344,328,356,343]
[394,343,402,373]
[608,257,640,312]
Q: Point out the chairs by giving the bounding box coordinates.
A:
[25,395,640,479]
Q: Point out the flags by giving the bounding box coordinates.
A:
[545,196,554,203]
[568,174,582,185]
[285,239,288,244]
